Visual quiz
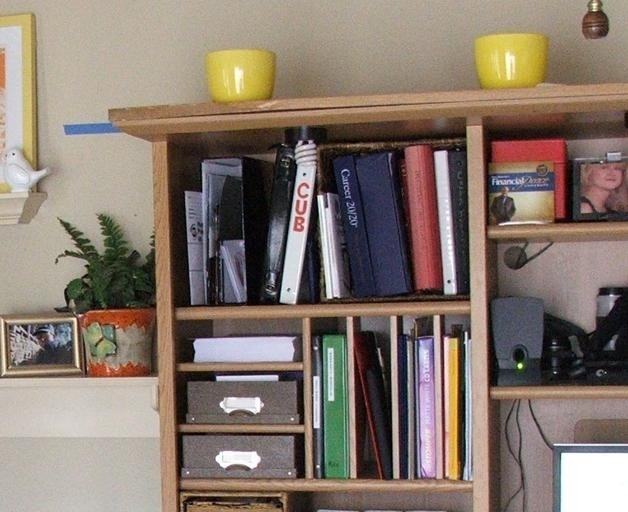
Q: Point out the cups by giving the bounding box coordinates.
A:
[206,47,276,100]
[474,31,549,90]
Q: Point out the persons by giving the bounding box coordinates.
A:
[490,186,516,225]
[20,327,71,363]
[580,162,624,213]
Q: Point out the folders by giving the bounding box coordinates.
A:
[322,331,347,480]
[259,143,470,305]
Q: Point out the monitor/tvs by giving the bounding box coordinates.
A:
[552,442,628,512]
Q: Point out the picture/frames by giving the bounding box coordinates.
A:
[0,13,40,194]
[0,313,86,378]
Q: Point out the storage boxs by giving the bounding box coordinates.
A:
[490,137,573,221]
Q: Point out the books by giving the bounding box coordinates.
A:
[216,374,295,381]
[180,146,472,307]
[487,161,557,226]
[312,328,474,482]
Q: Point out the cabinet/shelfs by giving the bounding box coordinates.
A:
[483,83,628,408]
[107,81,485,512]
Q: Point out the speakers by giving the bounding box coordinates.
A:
[489,295,545,386]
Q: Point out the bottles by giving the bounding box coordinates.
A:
[595,284,628,358]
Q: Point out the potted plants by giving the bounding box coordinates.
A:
[51,209,156,376]
